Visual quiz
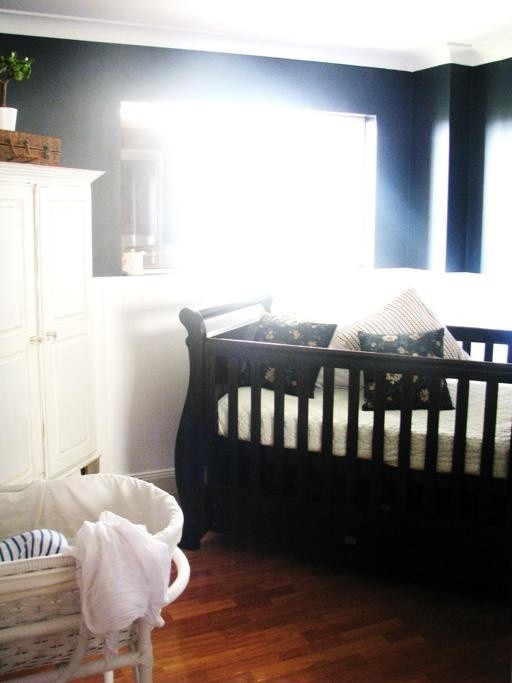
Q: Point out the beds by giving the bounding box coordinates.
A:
[0,474,190,683]
[174,292,511,613]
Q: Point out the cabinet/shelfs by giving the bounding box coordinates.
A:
[0,161,105,487]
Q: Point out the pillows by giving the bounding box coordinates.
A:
[240,285,471,412]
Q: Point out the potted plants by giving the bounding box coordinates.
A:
[0,50,34,132]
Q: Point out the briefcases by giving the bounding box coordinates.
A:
[0,129,62,166]
